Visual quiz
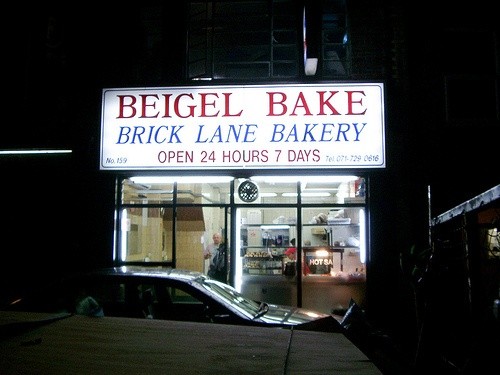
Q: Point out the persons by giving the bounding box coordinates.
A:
[205,233,225,266]
[284,238,312,274]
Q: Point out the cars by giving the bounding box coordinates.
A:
[73,266,344,330]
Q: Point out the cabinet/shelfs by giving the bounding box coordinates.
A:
[243,257,286,275]
